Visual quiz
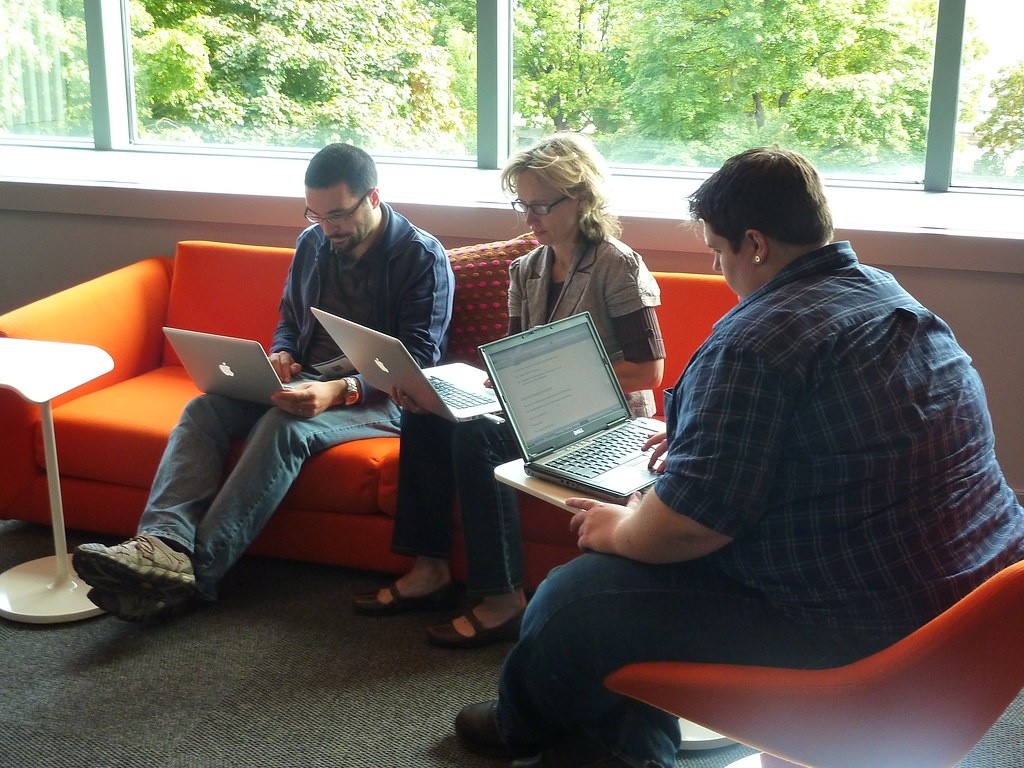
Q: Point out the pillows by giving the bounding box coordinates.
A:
[436,232,539,370]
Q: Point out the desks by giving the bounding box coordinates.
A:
[0,336,114,625]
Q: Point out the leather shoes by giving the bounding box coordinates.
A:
[510,728,634,767]
[454,698,527,758]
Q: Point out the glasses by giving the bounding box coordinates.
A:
[303,190,371,226]
[510,193,575,217]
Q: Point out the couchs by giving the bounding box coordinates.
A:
[0,241,739,589]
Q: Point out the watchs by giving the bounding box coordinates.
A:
[342,376,359,406]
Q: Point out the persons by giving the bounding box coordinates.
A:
[71,142,456,621]
[350,134,664,646]
[454,149,1022,768]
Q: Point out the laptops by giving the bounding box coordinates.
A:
[478,310,667,504]
[161,327,319,406]
[311,307,505,423]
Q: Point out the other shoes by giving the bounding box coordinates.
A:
[352,576,466,615]
[423,603,534,647]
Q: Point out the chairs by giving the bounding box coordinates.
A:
[605,560,1024,768]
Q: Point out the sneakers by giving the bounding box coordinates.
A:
[71,533,197,600]
[88,586,190,627]
